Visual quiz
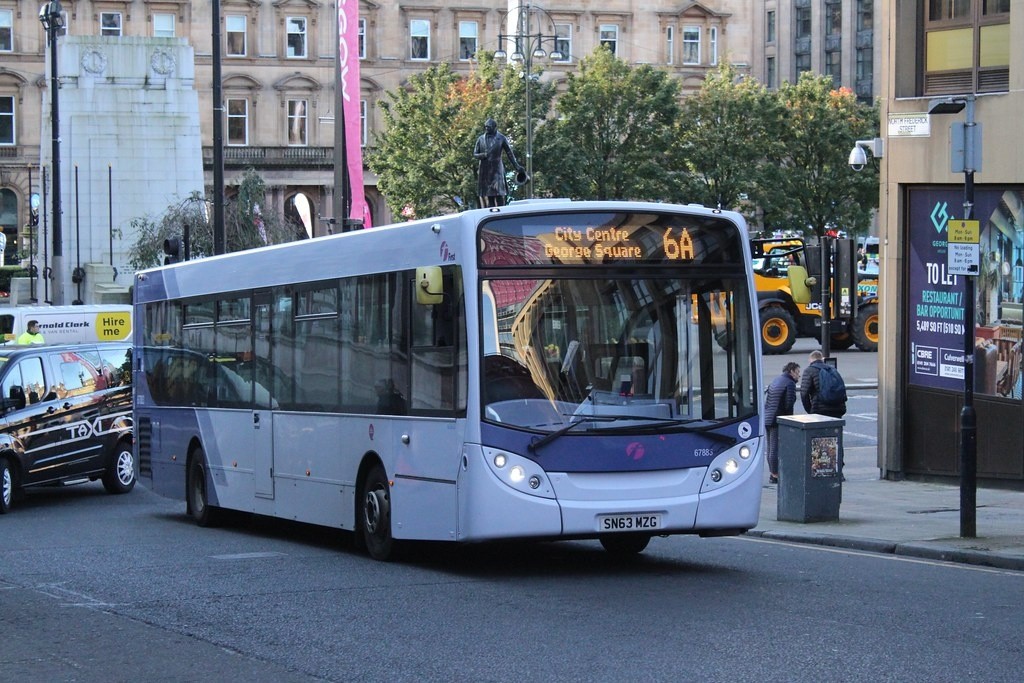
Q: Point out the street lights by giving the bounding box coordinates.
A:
[493,4,564,199]
[37,1,68,304]
[928,94,977,536]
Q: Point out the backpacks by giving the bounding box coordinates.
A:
[810,364,848,406]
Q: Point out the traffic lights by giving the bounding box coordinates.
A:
[163,238,183,265]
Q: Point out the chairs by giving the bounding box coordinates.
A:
[997,341,1021,398]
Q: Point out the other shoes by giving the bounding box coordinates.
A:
[841,473,846,482]
[769,474,778,483]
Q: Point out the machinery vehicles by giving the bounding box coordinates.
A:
[708,237,878,357]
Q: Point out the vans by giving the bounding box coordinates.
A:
[0,341,137,515]
[0,304,134,349]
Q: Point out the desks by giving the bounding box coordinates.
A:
[997,361,1007,381]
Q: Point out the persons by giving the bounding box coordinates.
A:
[800,351,847,483]
[17,320,44,344]
[857,247,867,271]
[474,119,524,208]
[762,362,801,484]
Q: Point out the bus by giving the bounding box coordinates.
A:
[132,196,816,564]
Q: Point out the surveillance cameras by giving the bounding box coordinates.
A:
[848,147,867,171]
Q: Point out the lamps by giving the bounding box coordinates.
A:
[1014,249,1023,268]
